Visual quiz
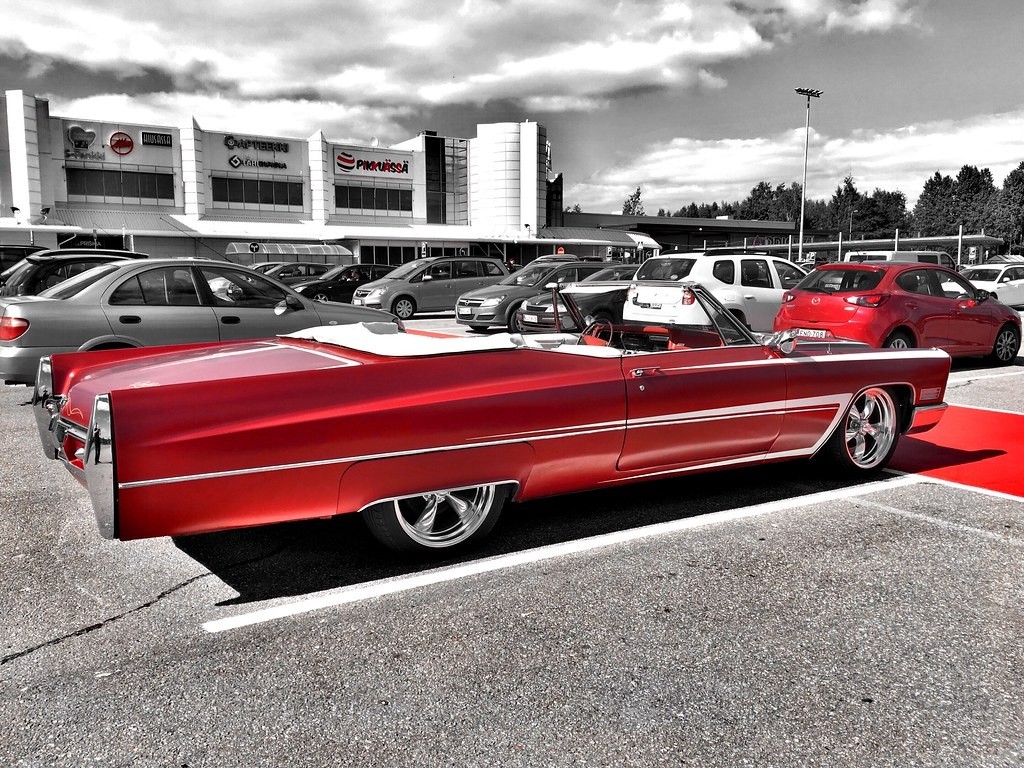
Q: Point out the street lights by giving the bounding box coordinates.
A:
[794,87,824,262]
[848,209,859,240]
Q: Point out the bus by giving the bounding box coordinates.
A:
[843,250,959,272]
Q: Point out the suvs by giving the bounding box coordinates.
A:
[621,249,808,333]
[350,255,510,320]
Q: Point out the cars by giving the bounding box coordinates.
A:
[31,281,950,558]
[0,244,150,298]
[454,257,625,333]
[515,263,643,334]
[247,262,290,274]
[775,262,817,289]
[263,261,335,286]
[0,258,405,406]
[769,258,1024,369]
[288,263,400,302]
[941,263,1024,306]
